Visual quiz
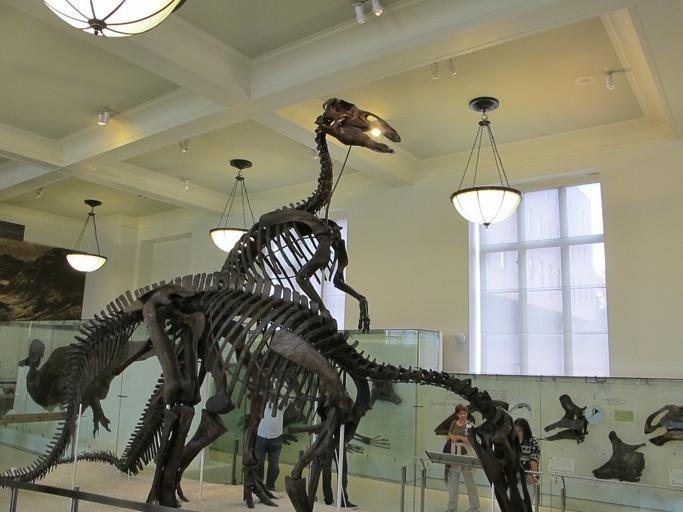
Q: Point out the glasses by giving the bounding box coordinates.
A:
[446,96,525,230]
[94,110,110,126]
[63,200,108,275]
[429,57,455,81]
[604,70,614,92]
[350,0,384,26]
[208,158,257,255]
[40,0,185,39]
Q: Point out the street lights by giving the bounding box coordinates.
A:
[442,438,468,455]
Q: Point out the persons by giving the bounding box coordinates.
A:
[257,380,292,492]
[320,417,358,511]
[513,418,541,505]
[447,404,481,512]
